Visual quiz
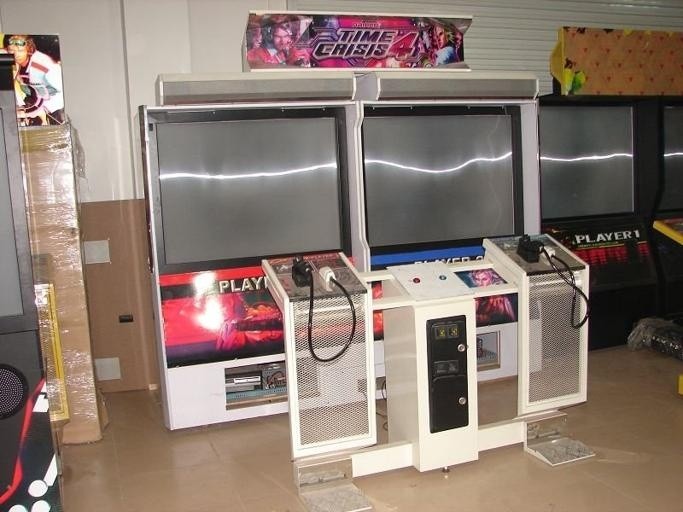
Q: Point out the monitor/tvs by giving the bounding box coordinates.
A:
[0,90,40,335]
[537,101,639,223]
[653,101,683,217]
[147,106,352,273]
[361,103,525,256]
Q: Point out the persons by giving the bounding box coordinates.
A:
[5,36,64,126]
[470,269,513,324]
[247,24,308,66]
[430,22,456,66]
